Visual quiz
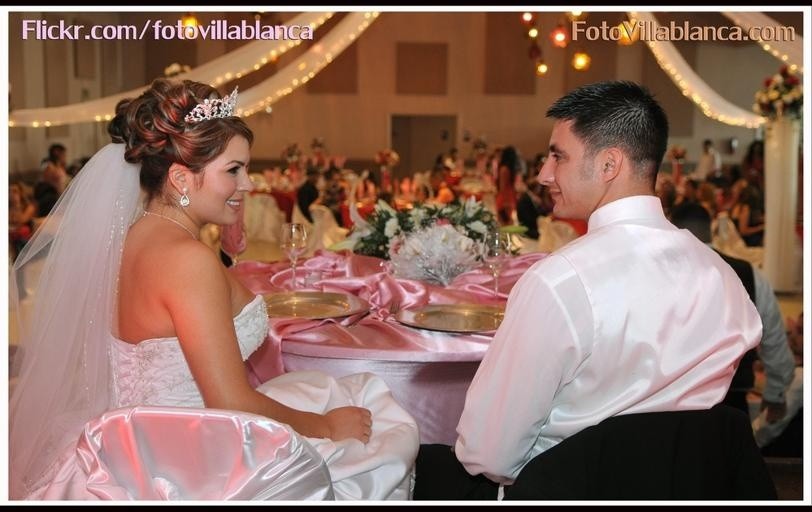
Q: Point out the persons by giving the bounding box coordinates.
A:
[671,205,796,461]
[454,79,763,499]
[8,78,420,501]
[9,138,763,247]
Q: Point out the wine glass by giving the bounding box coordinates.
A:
[479,232,511,302]
[279,223,308,291]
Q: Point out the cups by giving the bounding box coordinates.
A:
[302,271,324,291]
[244,173,302,194]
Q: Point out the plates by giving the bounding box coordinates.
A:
[259,290,371,321]
[394,303,505,334]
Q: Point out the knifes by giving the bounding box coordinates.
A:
[345,307,371,330]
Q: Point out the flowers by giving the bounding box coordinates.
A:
[753,64,804,117]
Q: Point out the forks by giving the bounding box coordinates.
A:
[382,301,400,322]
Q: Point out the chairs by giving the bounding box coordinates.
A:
[8,153,780,251]
[75,405,335,503]
[503,405,782,511]
